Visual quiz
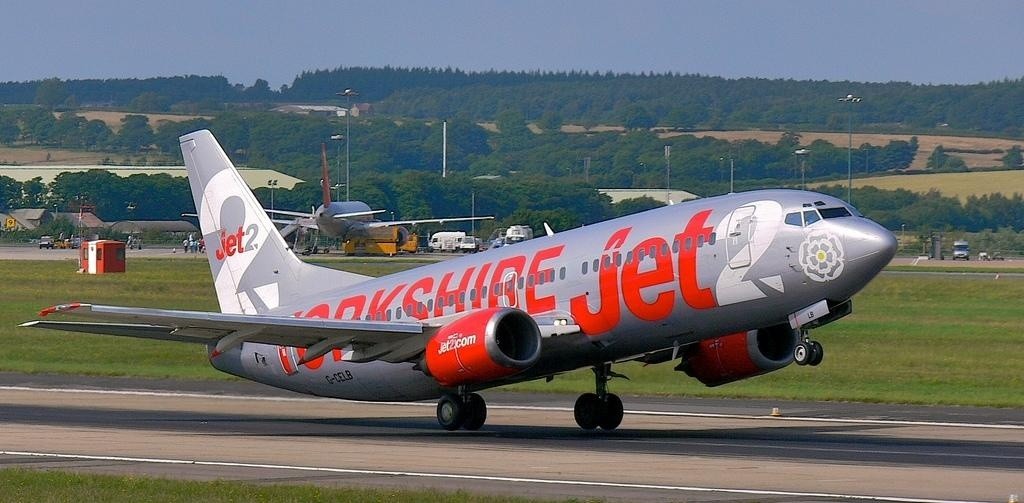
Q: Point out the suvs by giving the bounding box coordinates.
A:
[39,236,55,249]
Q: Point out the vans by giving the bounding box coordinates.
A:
[504,224,534,247]
[951,239,970,261]
[428,231,466,253]
[460,235,480,254]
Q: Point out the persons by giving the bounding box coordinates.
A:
[125,233,143,251]
[171,232,205,254]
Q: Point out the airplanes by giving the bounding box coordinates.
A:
[180,140,495,257]
[16,124,900,438]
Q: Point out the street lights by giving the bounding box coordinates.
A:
[794,148,814,191]
[329,134,346,204]
[339,87,361,205]
[838,93,866,205]
[267,179,278,219]
[662,143,672,207]
